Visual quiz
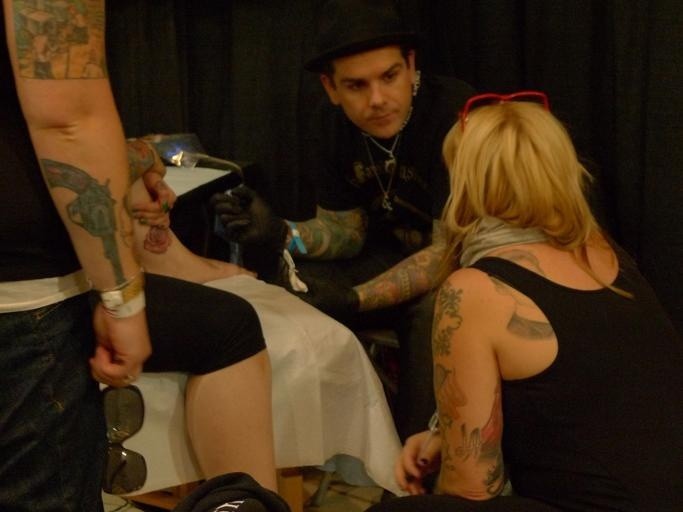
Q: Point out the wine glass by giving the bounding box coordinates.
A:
[124,373,134,389]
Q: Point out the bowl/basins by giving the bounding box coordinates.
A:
[352,108,413,215]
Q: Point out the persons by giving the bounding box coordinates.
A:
[121,137,282,500]
[0,0,154,510]
[205,35,602,426]
[368,99,679,510]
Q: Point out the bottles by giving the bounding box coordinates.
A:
[310,6,421,68]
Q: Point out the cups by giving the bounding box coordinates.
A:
[97,384,148,496]
[459,90,550,133]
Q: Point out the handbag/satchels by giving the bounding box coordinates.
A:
[284,217,309,263]
[90,270,145,320]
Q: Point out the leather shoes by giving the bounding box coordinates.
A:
[212,185,288,248]
[309,279,362,318]
